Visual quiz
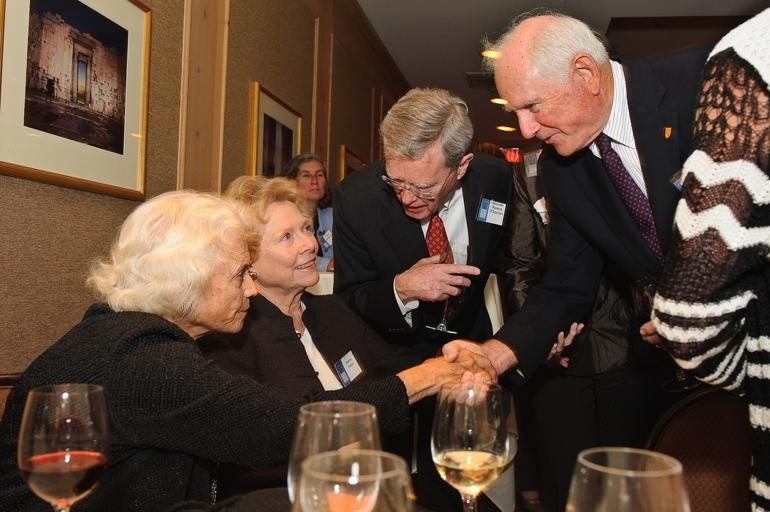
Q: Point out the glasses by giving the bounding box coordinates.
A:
[378,153,464,201]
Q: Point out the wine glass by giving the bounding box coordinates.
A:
[15,382,112,512]
[429,377,524,511]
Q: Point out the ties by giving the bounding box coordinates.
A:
[592,135,662,265]
[426,215,454,321]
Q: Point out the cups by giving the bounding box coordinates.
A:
[295,446,420,511]
[557,447,698,510]
[286,397,383,512]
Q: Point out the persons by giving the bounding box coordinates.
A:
[2,155,496,512]
[332,7,769,512]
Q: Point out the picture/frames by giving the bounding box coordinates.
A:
[1,1,155,204]
[339,142,367,182]
[250,78,306,183]
